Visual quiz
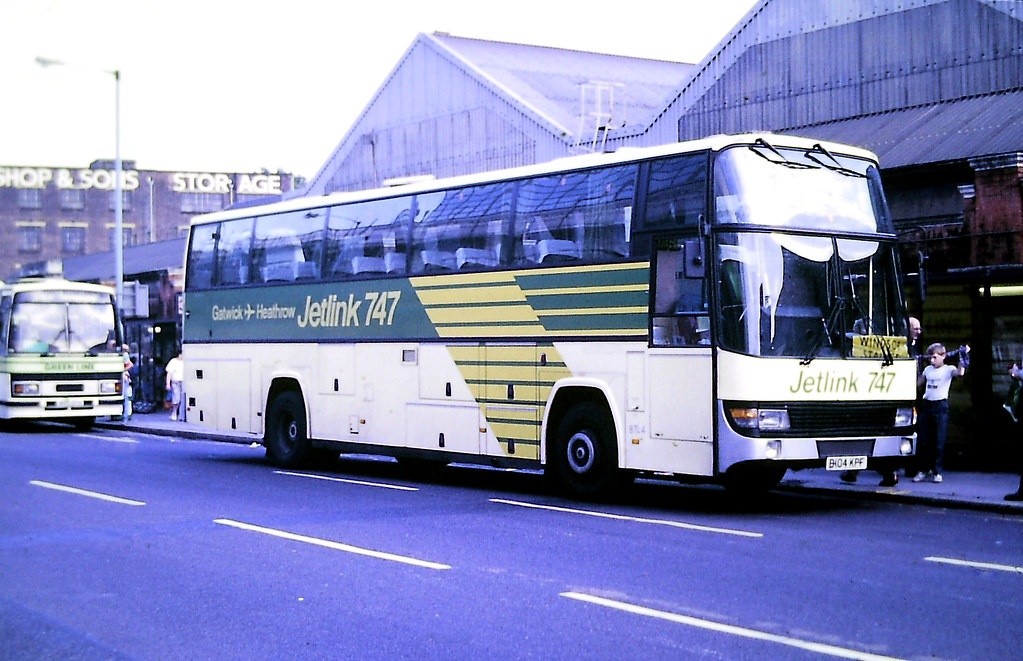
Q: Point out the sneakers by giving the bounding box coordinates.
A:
[932,475,943,483]
[912,472,926,483]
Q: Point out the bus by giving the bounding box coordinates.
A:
[177,129,919,498]
[1,281,126,434]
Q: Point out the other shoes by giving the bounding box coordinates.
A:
[879,473,898,487]
[1004,493,1023,501]
[840,471,857,483]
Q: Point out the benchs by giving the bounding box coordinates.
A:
[352,256,386,275]
[384,252,425,274]
[263,263,294,282]
[537,238,580,264]
[294,262,321,280]
[239,266,250,285]
[456,248,498,269]
[421,250,458,273]
[494,242,539,265]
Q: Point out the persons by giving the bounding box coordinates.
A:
[106,340,135,420]
[905,317,968,483]
[164,351,183,420]
[1001,363,1023,500]
